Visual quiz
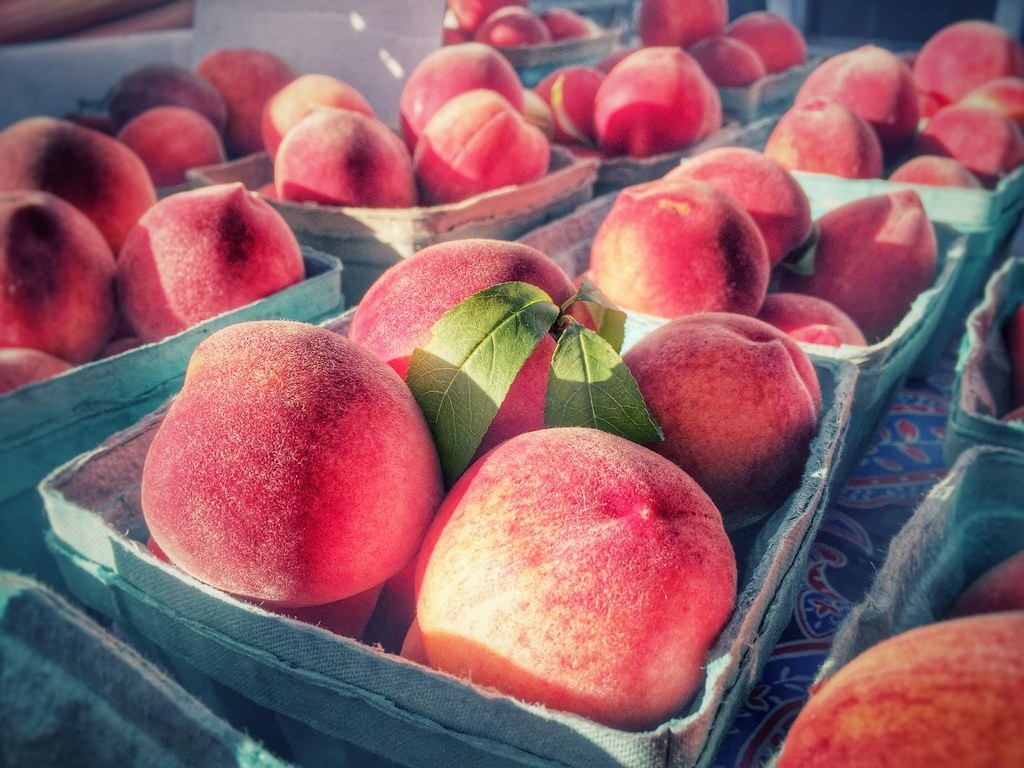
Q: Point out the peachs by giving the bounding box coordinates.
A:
[0,0,1024,768]
